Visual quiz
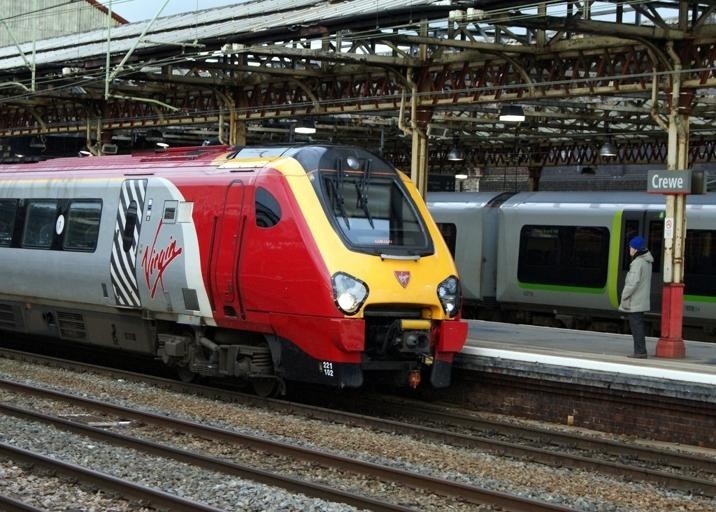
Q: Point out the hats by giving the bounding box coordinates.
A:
[628,235,647,251]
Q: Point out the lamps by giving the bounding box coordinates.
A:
[598,136,618,157]
[499,105,525,122]
[448,148,465,161]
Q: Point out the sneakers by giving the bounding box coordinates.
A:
[625,351,648,358]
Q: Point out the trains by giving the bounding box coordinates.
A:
[1,138,472,399]
[424,190,716,333]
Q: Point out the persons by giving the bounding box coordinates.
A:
[617,235,655,360]
